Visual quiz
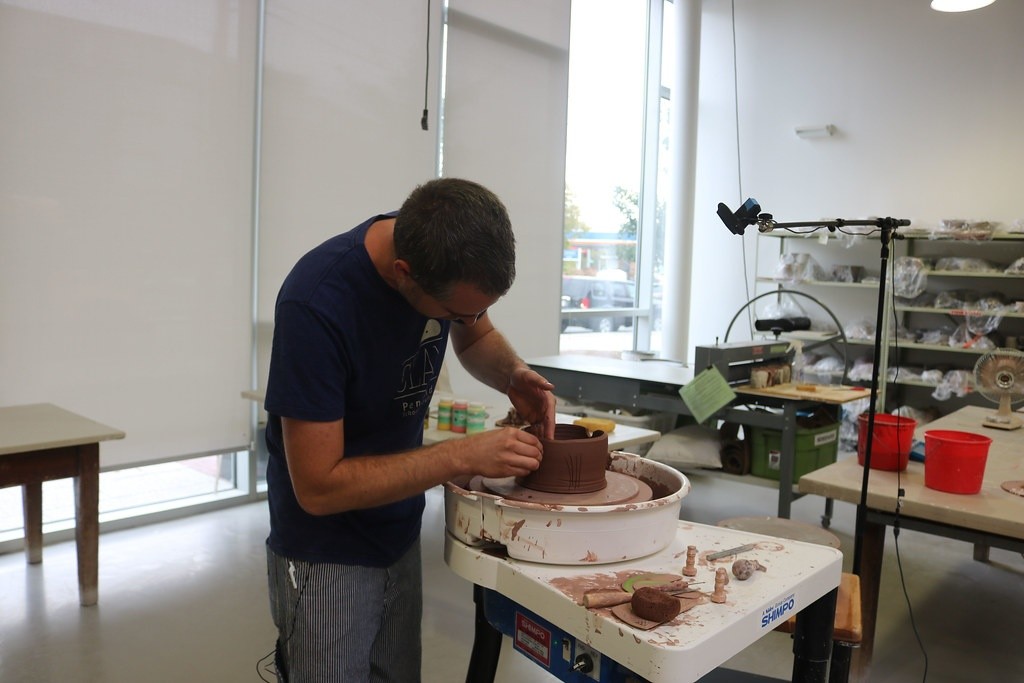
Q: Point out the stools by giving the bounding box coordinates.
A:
[773,572,861,683]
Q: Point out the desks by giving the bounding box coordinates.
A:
[523,353,881,526]
[800,406,1024,683]
[0,403,125,607]
[240,391,661,452]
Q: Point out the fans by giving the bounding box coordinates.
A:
[973,347,1024,431]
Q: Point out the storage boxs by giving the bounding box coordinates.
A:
[750,422,844,483]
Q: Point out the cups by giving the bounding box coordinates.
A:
[750,362,792,389]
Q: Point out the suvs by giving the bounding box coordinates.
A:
[559,274,636,333]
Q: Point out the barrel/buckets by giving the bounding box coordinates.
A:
[855,414,918,472]
[923,429,993,495]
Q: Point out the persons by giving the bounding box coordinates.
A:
[264,177,556,683]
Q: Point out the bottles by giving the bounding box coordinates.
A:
[436,396,485,436]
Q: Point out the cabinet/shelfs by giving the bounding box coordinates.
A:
[751,224,1023,446]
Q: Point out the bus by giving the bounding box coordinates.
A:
[563,237,637,278]
